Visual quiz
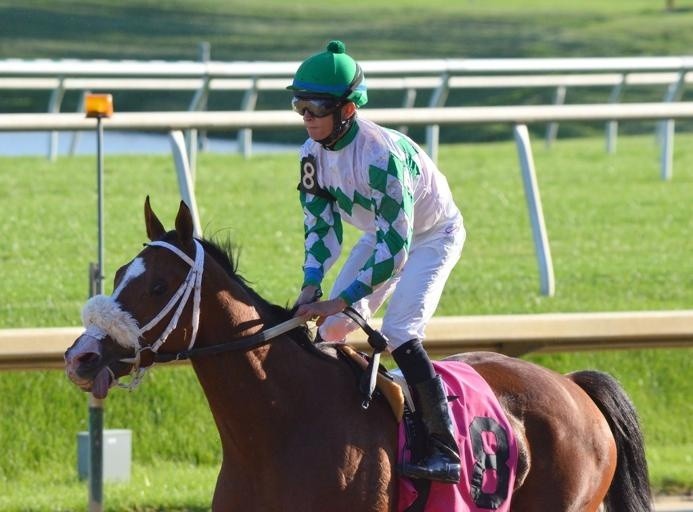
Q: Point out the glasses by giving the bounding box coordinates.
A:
[292,64,363,117]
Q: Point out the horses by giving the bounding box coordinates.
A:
[62,192,655,512]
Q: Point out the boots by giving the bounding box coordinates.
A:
[403,374,461,483]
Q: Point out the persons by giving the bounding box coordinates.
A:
[290,36,467,484]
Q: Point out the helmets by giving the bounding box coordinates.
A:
[287,41,368,108]
